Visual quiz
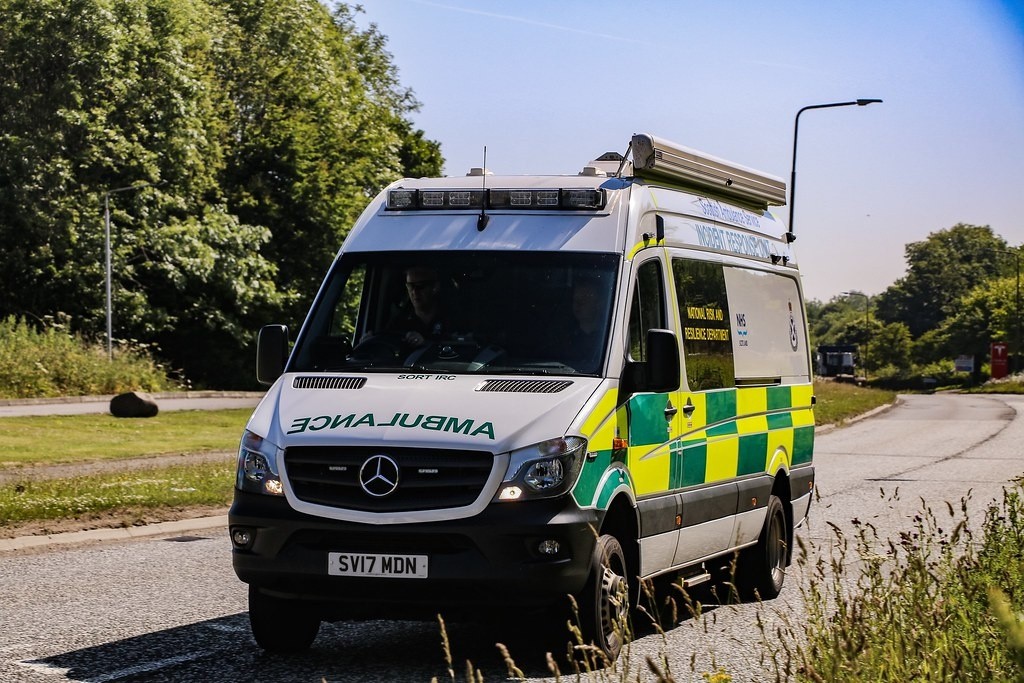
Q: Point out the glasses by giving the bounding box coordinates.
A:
[405,280,427,291]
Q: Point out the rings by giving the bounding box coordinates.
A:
[414,339,417,342]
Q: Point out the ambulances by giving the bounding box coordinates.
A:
[226,134,814,675]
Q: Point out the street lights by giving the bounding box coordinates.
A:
[840,291,869,380]
[788,98,883,233]
[100,178,169,367]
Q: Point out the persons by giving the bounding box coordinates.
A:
[358,265,454,360]
[532,275,609,373]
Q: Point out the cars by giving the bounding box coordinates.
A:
[836,374,867,386]
